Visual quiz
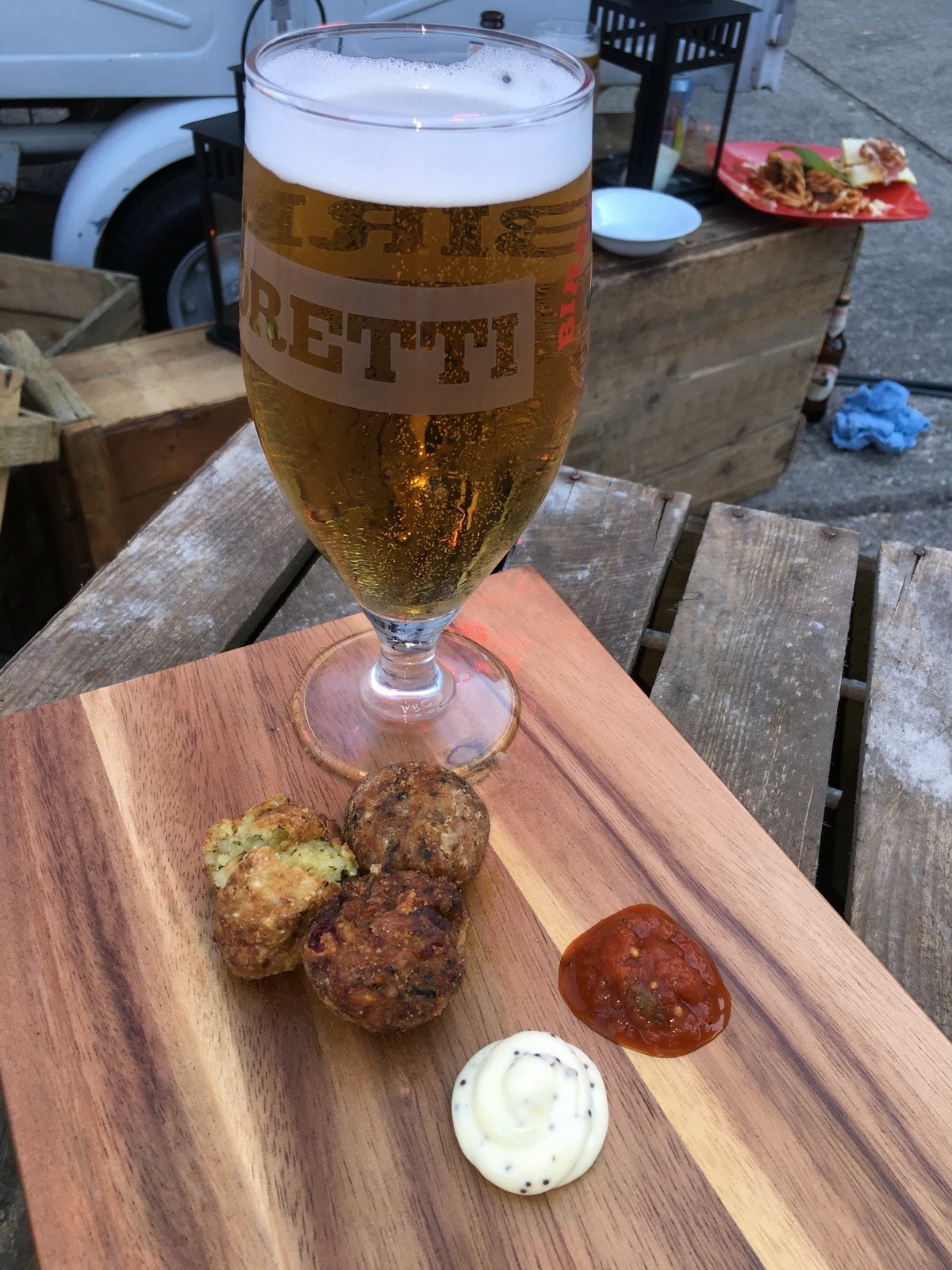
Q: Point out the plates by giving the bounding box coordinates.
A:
[717,141,932,222]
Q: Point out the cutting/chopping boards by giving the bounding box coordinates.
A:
[0,564,951,1270]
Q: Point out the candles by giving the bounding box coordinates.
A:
[615,137,680,197]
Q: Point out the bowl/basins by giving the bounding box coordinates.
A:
[590,185,702,257]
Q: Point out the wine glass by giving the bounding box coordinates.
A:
[232,23,594,788]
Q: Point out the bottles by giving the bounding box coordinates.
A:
[802,293,851,425]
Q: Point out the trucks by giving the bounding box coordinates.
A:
[1,0,799,335]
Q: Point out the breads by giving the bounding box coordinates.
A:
[841,137,917,189]
[198,760,491,1032]
